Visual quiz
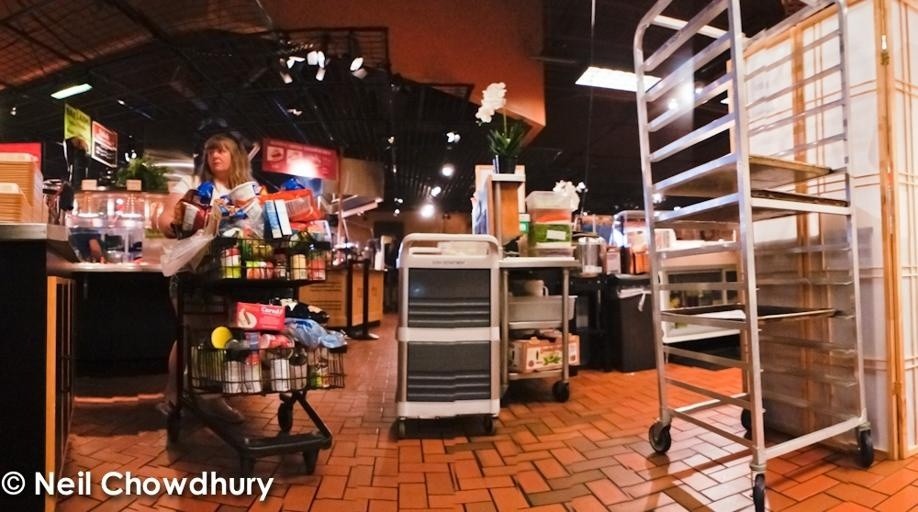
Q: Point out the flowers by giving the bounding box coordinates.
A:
[473,82,531,153]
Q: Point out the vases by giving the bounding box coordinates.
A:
[497,155,516,174]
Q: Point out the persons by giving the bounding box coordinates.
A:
[156,134,260,423]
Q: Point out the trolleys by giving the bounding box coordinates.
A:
[173,242,327,469]
[498,215,576,398]
[395,236,502,441]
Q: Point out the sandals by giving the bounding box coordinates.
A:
[155,393,193,417]
[198,396,244,424]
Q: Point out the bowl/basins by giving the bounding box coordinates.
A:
[64,208,144,262]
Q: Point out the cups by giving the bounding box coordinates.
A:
[514,280,548,297]
